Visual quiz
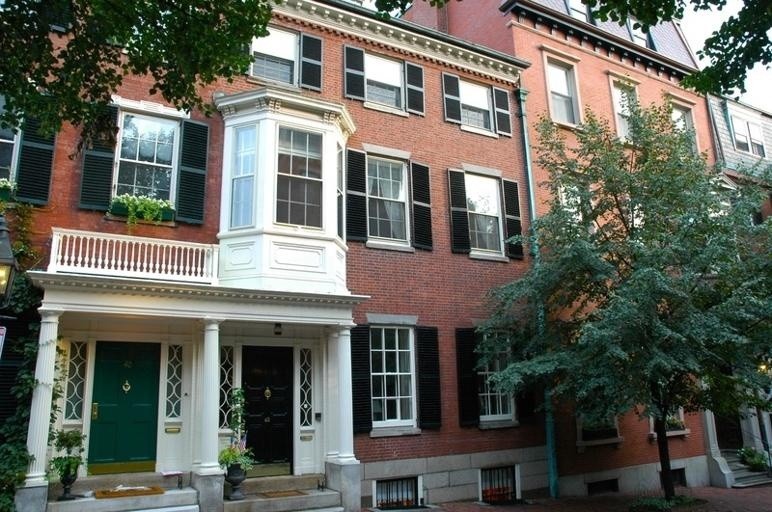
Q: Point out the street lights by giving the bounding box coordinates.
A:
[0,214,18,314]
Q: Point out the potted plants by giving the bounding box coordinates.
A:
[108,193,176,230]
[218,387,253,501]
[737,447,766,471]
[45,427,87,501]
[0,177,12,201]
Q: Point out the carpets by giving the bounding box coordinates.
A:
[95,484,164,498]
[256,489,308,498]
